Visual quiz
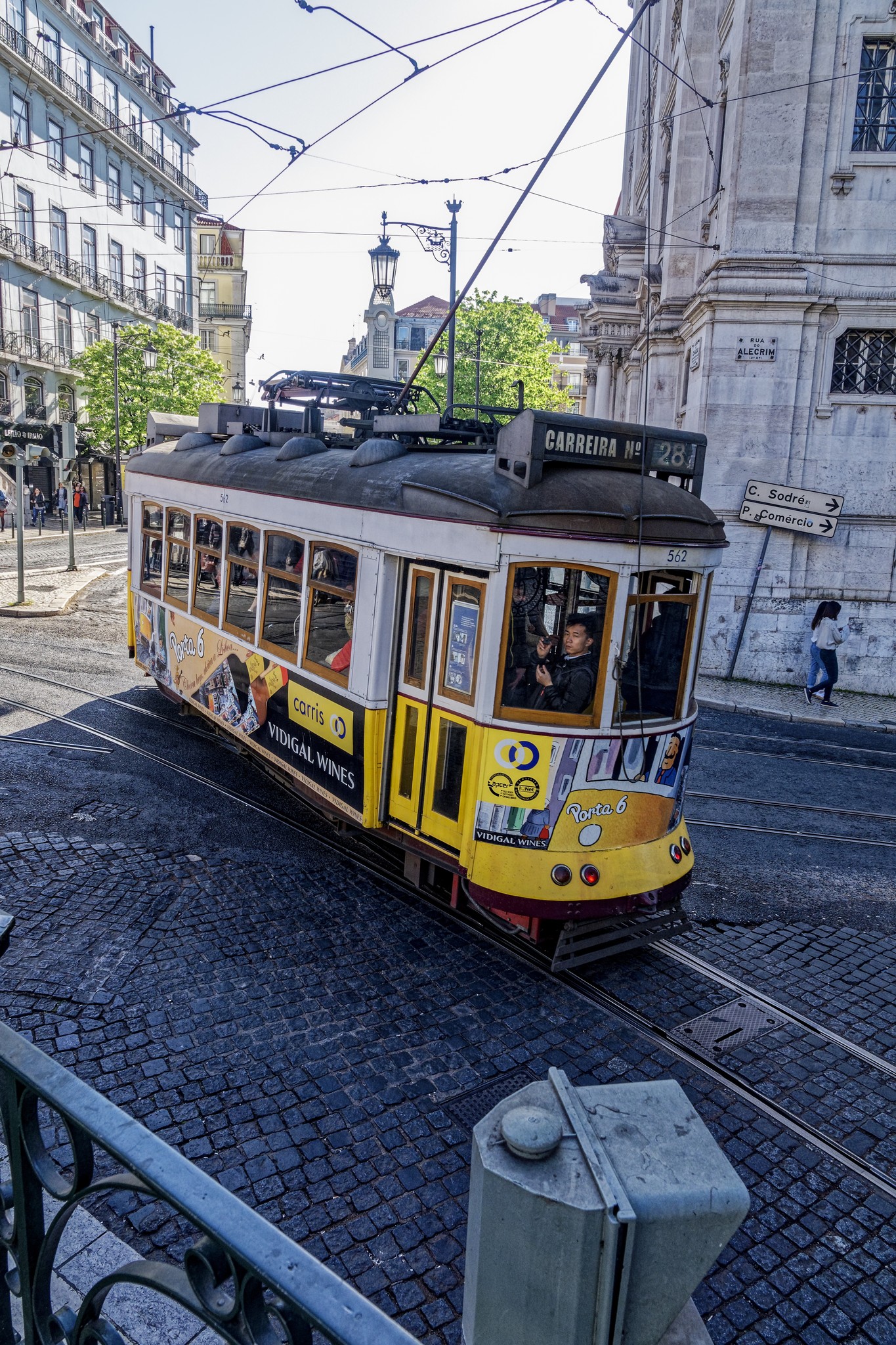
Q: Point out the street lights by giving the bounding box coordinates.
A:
[367,193,463,418]
[110,321,159,522]
[432,327,485,421]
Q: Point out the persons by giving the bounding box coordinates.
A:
[55,483,68,523]
[30,487,46,527]
[72,482,89,525]
[0,490,7,532]
[804,601,851,708]
[807,601,829,700]
[622,588,691,715]
[146,509,548,705]
[522,613,598,714]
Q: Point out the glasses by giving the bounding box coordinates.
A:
[80,488,84,489]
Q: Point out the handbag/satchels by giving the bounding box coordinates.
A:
[54,499,59,506]
[3,496,12,508]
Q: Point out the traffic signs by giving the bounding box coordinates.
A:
[739,500,838,538]
[744,479,844,517]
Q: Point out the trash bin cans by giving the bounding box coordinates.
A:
[100,495,115,526]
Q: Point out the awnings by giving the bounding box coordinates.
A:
[38,447,63,468]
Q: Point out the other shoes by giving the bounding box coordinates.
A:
[30,523,35,526]
[811,692,817,696]
[211,585,219,590]
[816,690,825,699]
[78,522,82,525]
[41,524,45,527]
[804,687,813,704]
[821,701,839,708]
[0,529,5,532]
[59,518,62,521]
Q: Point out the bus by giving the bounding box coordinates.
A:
[116,367,730,976]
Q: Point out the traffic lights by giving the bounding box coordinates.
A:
[26,445,50,461]
[59,458,76,483]
[0,443,17,459]
